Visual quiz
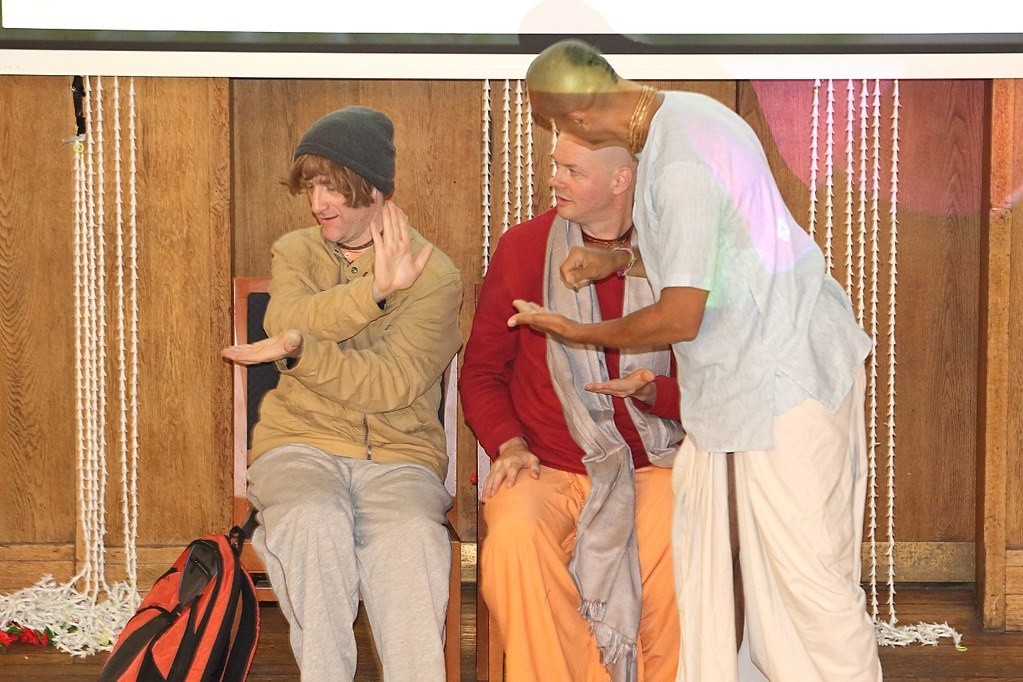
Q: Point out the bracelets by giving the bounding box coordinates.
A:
[610,247,638,276]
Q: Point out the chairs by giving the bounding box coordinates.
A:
[228,277,507,681]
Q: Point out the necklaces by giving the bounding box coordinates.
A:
[335,229,385,259]
[581,232,632,246]
[626,84,659,155]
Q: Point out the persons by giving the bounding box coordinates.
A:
[507,39,892,682]
[455,129,681,682]
[219,105,466,682]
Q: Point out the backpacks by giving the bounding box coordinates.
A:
[98,524,261,682]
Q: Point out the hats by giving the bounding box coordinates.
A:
[294,105,397,194]
